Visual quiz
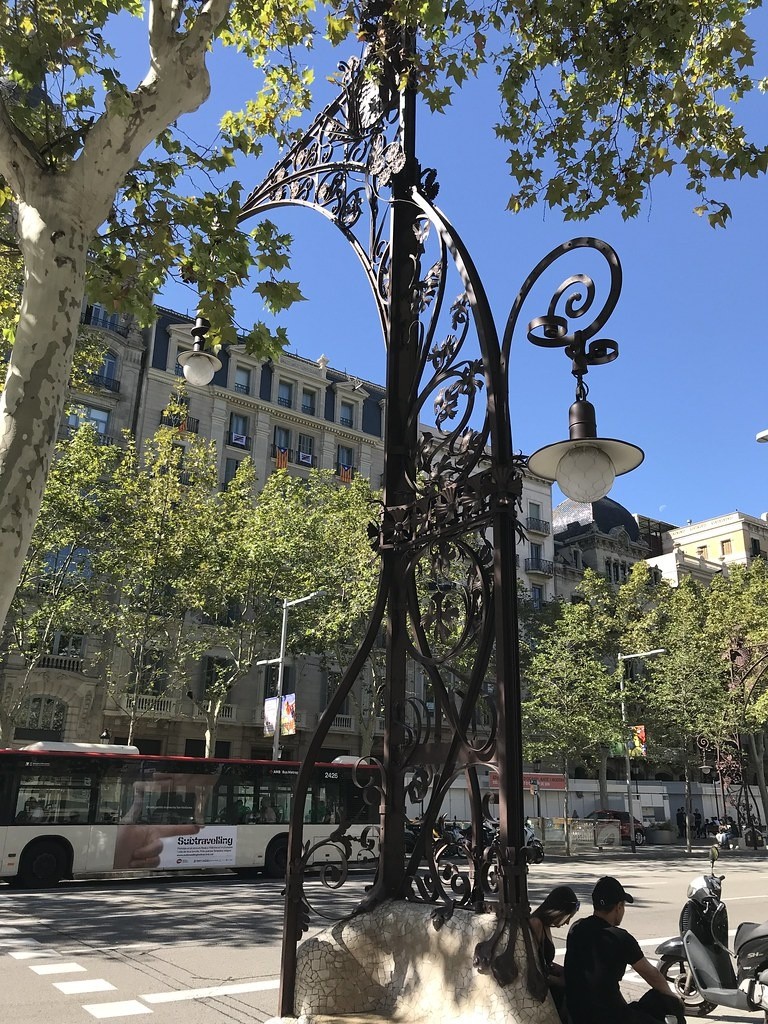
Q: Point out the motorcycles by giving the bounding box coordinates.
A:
[404,814,545,865]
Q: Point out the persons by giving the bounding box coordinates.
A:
[676,806,738,840]
[562,875,688,1024]
[149,788,340,826]
[571,810,579,818]
[527,885,581,1010]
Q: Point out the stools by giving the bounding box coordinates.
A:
[734,917,768,955]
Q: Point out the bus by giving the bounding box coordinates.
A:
[0,737,387,887]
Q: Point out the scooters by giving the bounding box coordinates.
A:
[654,846,768,1023]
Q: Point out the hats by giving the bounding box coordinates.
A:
[590,874,634,910]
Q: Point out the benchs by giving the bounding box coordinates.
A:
[728,825,747,849]
[292,898,561,1024]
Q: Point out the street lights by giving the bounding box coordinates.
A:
[178,6,651,921]
[617,648,668,853]
[696,731,728,826]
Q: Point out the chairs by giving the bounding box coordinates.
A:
[13,803,333,821]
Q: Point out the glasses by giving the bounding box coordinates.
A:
[559,898,583,912]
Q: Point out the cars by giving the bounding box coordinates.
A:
[572,810,646,845]
